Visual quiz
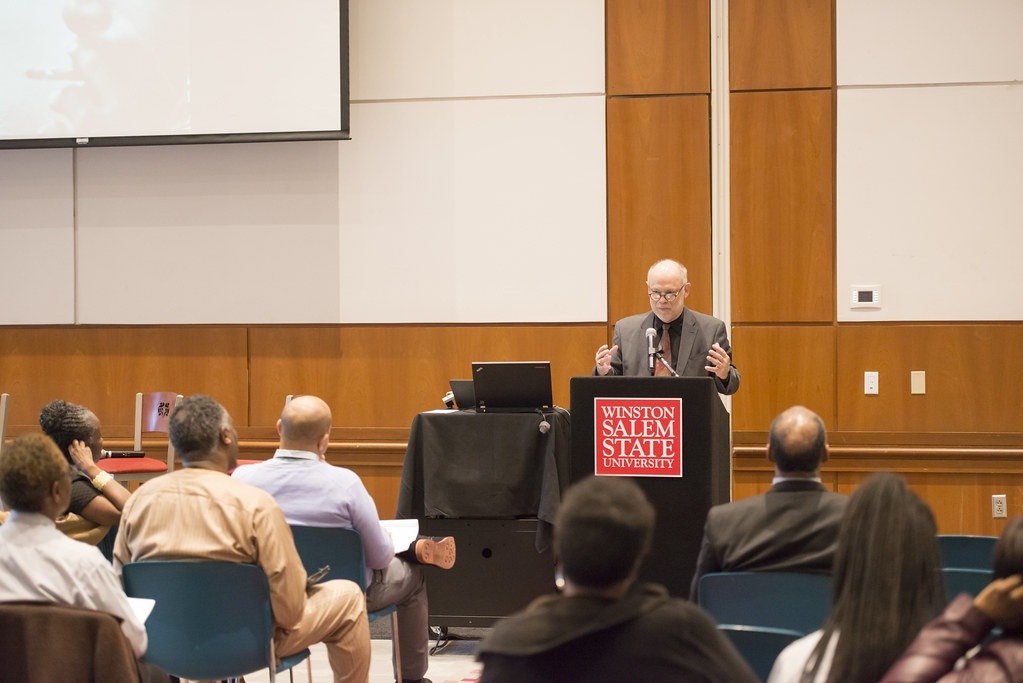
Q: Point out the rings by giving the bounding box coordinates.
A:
[598,361,601,364]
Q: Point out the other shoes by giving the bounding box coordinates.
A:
[395,678,432,683]
[407,534,457,570]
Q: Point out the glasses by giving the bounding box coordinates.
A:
[648,282,688,302]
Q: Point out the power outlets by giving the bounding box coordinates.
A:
[991,495,1006,518]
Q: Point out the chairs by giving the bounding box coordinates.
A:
[287,524,400,683]
[121,560,311,683]
[95,391,183,481]
[695,533,1000,683]
[0,604,180,683]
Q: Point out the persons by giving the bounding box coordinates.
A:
[690,404,852,606]
[0,433,148,659]
[592,257,741,395]
[882,513,1023,683]
[474,474,760,683]
[229,394,455,683]
[765,473,949,683]
[111,395,371,683]
[40,400,132,527]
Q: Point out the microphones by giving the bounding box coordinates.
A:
[100,448,145,459]
[645,327,657,372]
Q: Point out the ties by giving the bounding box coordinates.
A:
[654,323,672,377]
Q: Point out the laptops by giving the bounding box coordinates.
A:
[471,361,554,414]
[449,379,476,410]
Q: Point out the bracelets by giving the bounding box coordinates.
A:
[91,471,112,491]
